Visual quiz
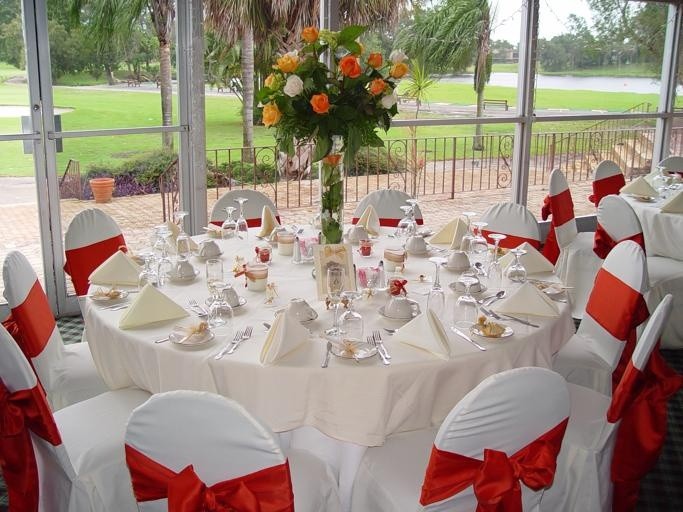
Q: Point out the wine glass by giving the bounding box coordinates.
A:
[139,197,527,334]
[643,165,683,201]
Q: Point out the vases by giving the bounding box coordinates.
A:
[319,133,344,240]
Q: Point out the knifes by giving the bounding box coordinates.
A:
[98,301,126,310]
[320,341,331,368]
[451,326,487,351]
[503,314,539,328]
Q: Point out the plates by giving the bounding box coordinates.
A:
[91,286,129,301]
[329,340,378,360]
[470,322,516,339]
[168,328,214,346]
[531,281,567,295]
[632,196,655,201]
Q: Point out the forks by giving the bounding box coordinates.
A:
[368,330,391,366]
[214,326,252,361]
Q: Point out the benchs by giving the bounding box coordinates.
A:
[217,82,236,93]
[128,79,141,87]
[482,100,509,111]
[397,93,419,107]
[156,80,161,89]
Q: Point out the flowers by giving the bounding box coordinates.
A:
[255,25,400,245]
